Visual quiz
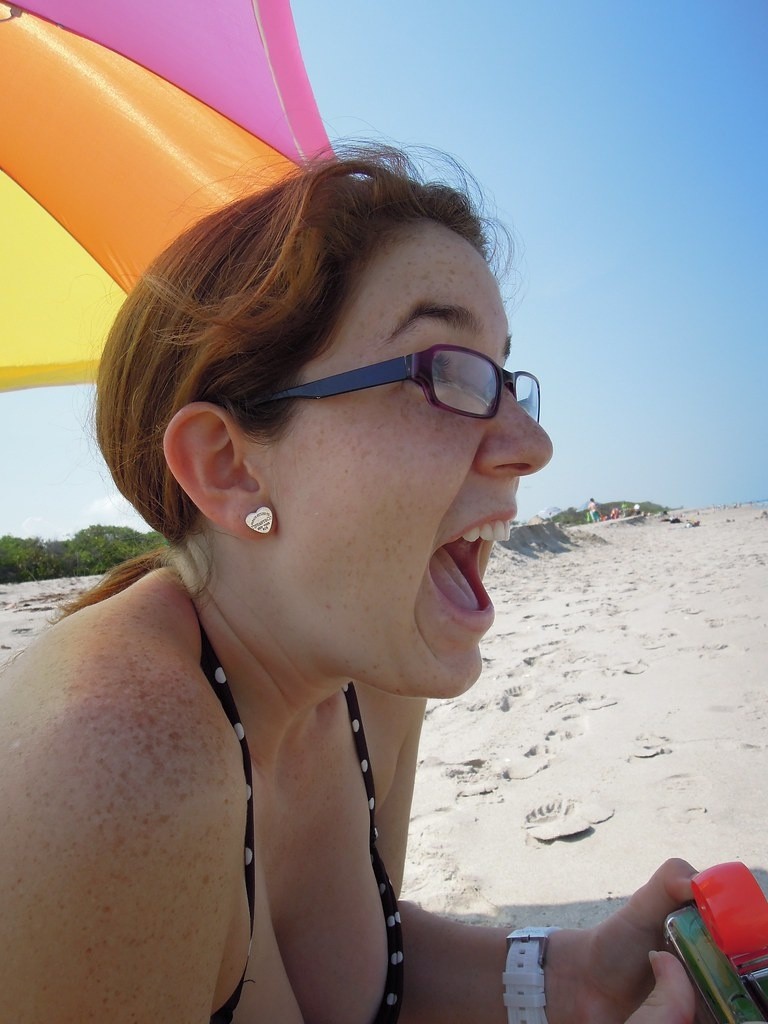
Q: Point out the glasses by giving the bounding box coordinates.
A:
[237,344,541,426]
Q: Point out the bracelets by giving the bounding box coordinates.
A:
[501,925,560,1023]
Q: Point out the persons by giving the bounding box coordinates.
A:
[586,495,639,524]
[0,135,702,1024]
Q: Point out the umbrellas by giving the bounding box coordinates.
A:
[0,0,338,397]
